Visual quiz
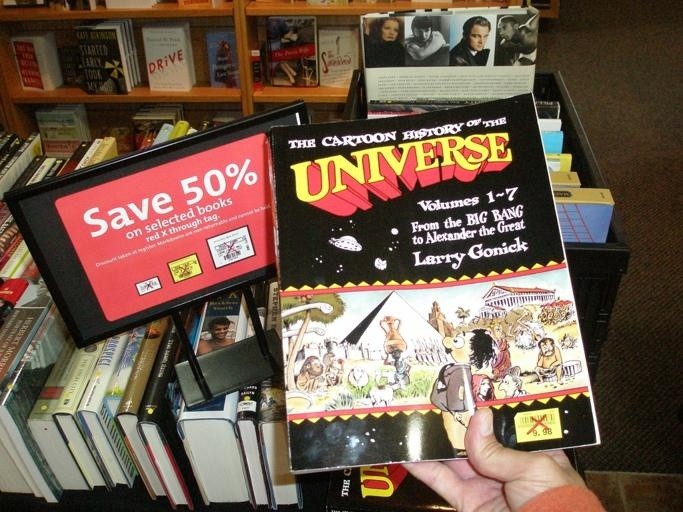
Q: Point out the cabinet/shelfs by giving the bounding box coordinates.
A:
[0,0,530,151]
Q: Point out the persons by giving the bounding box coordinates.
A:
[498,365,526,397]
[402,407,605,512]
[198,317,233,355]
[321,340,343,384]
[368,17,404,67]
[498,11,540,66]
[384,349,411,390]
[450,15,492,65]
[430,328,499,456]
[296,356,323,391]
[535,337,562,383]
[473,332,510,401]
[407,16,446,60]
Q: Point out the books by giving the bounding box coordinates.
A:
[267,15,319,87]
[527,102,616,243]
[3,0,44,9]
[327,449,588,512]
[0,122,306,510]
[205,28,241,88]
[107,0,153,9]
[211,111,242,127]
[36,104,93,160]
[319,24,360,89]
[363,6,540,100]
[77,19,140,95]
[12,31,63,91]
[269,92,601,473]
[143,21,197,92]
[131,104,184,147]
[252,40,266,89]
